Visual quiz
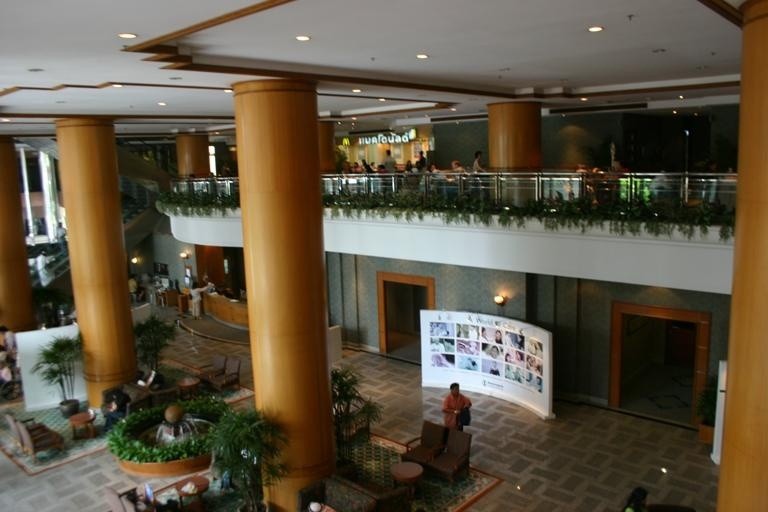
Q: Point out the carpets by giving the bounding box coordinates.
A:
[138,423,506,511]
[0,355,254,479]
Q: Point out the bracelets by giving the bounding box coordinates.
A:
[453,409,457,415]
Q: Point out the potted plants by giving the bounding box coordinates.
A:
[23,329,88,419]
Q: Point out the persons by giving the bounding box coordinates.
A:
[428,321,544,394]
[136,371,161,389]
[441,383,473,431]
[401,151,466,185]
[103,387,129,430]
[308,495,336,512]
[190,281,214,320]
[24,216,76,330]
[0,325,23,400]
[574,156,737,195]
[620,487,648,512]
[128,273,144,301]
[352,149,396,177]
[473,151,485,177]
[187,160,233,183]
[127,492,179,512]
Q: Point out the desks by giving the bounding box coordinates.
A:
[126,283,179,309]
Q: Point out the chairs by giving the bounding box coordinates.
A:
[5,350,244,462]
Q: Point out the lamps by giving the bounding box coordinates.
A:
[491,293,508,307]
